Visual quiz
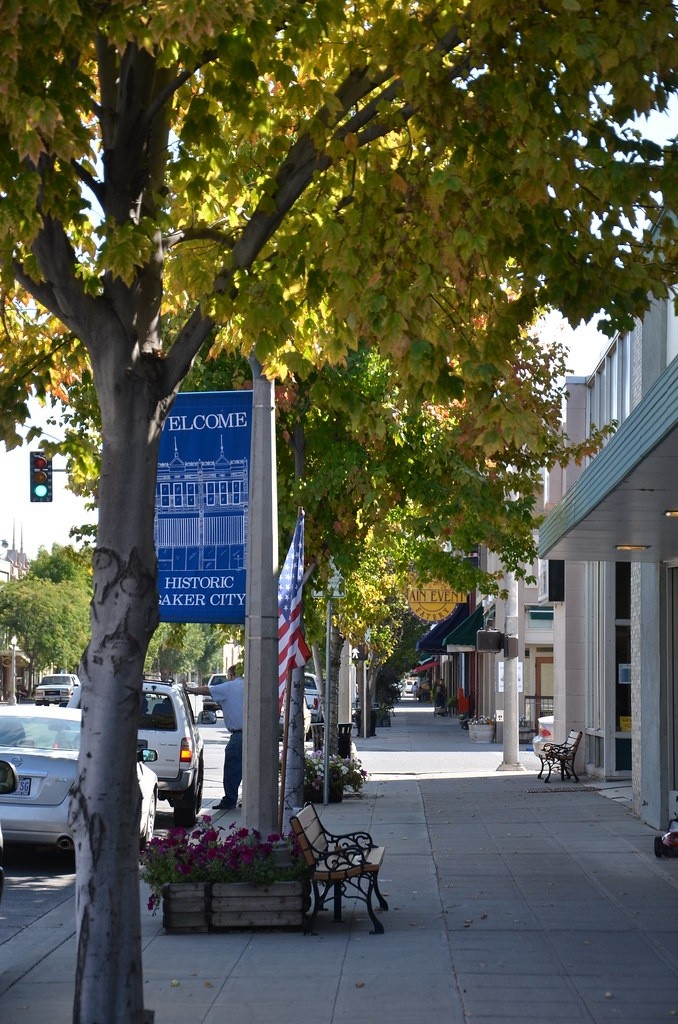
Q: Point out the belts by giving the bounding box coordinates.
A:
[233,730,242,733]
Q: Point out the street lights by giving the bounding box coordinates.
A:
[8,635,19,705]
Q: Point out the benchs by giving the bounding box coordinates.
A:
[537,729,583,783]
[289,801,389,936]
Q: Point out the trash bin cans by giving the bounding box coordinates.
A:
[309,722,353,804]
[352,707,378,737]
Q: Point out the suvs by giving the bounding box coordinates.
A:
[52,674,217,827]
[302,671,326,735]
[32,674,81,707]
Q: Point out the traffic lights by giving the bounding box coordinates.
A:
[352,644,368,661]
[29,450,53,503]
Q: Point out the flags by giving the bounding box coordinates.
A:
[278,506,311,837]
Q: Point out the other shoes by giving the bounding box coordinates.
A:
[212,802,237,809]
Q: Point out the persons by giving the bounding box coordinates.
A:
[181,665,244,809]
[411,682,417,697]
[434,679,444,707]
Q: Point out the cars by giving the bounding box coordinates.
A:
[278,686,314,743]
[172,671,230,724]
[530,716,554,760]
[0,760,22,905]
[394,679,417,694]
[1,703,161,866]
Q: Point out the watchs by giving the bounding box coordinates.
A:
[185,687,189,689]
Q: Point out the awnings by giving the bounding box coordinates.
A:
[409,656,440,677]
[415,594,484,655]
